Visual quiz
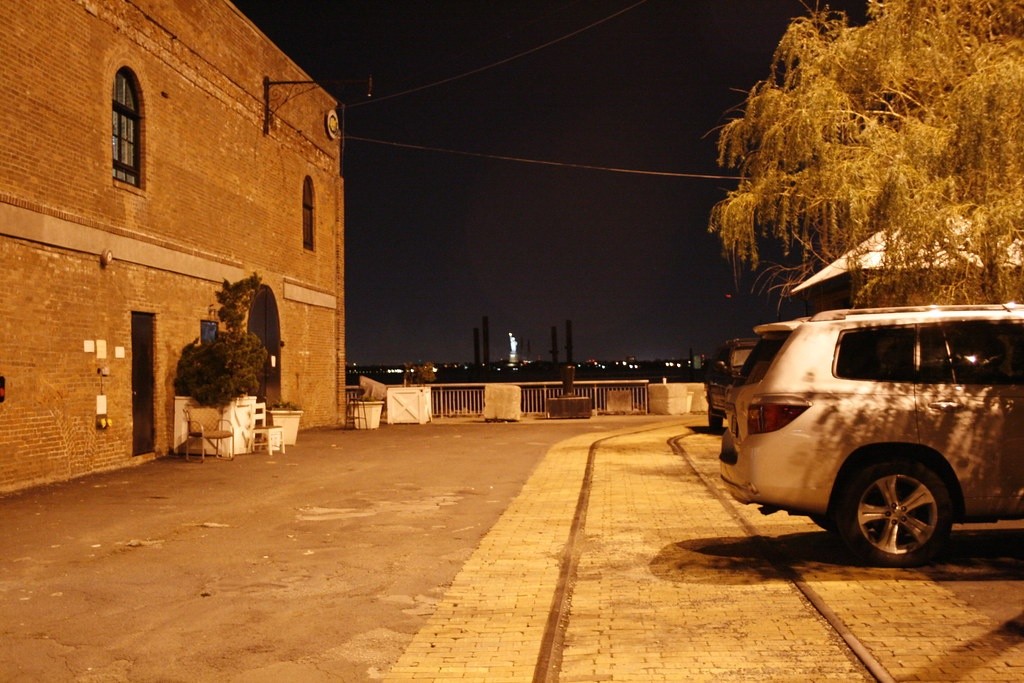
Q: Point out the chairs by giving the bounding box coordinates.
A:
[184,405,235,463]
[247,403,285,455]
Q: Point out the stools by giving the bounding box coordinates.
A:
[344,388,366,430]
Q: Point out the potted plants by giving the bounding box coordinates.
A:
[265,402,303,447]
[349,396,385,430]
[387,363,435,425]
[171,274,269,456]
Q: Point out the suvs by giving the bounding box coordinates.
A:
[719,303,1024,566]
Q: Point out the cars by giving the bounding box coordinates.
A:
[707,338,760,431]
